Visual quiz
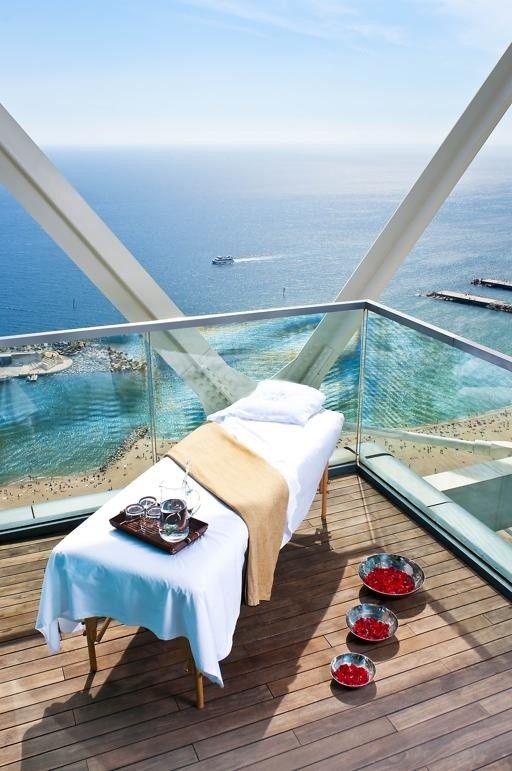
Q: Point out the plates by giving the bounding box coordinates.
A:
[110,510,208,553]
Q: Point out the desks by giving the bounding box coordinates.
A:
[54,410,345,711]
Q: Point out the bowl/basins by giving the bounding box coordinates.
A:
[358,553,425,597]
[329,652,377,688]
[345,603,399,643]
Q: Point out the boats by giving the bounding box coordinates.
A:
[212,255,234,264]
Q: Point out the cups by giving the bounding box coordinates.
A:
[125,496,160,536]
[157,480,201,543]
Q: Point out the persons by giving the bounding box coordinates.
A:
[336,409,511,473]
[0,423,165,503]
